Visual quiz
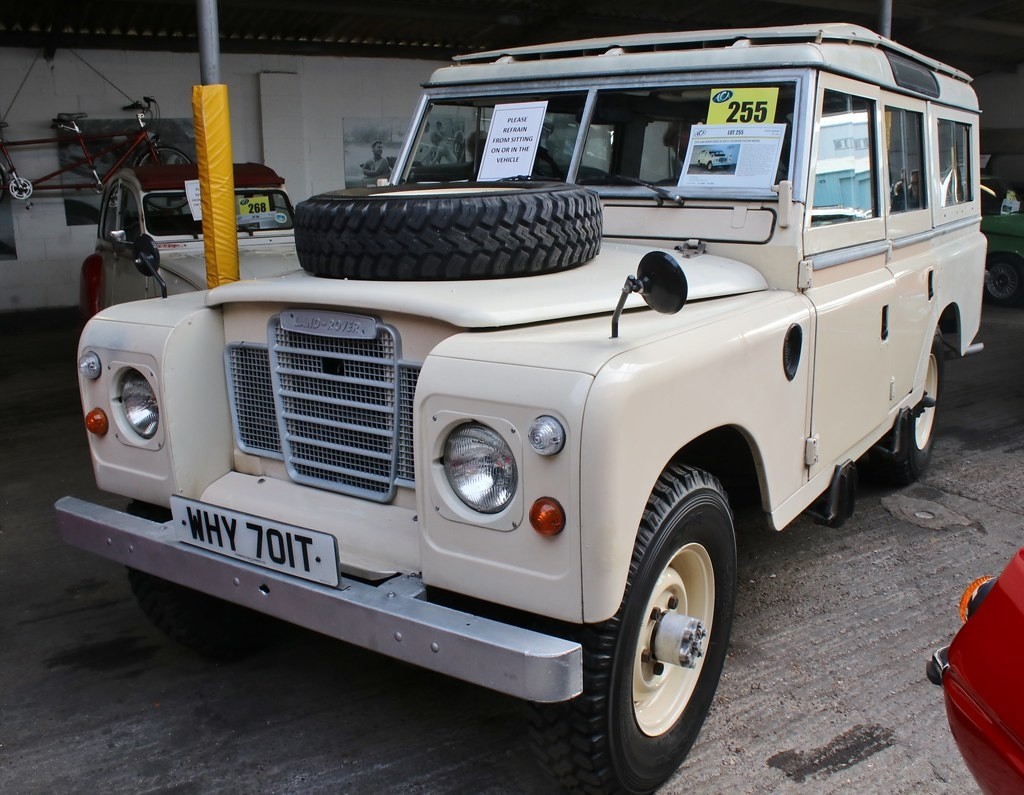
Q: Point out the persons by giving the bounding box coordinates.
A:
[891,169,918,211]
[662,114,788,185]
[422,120,465,163]
[363,141,388,177]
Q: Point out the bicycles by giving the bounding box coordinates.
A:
[0,97,194,212]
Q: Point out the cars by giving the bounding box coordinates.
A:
[924,545,1024,794]
[78,165,304,326]
[980,174,1024,309]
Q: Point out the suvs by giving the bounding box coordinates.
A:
[55,22,990,795]
[697,149,732,171]
